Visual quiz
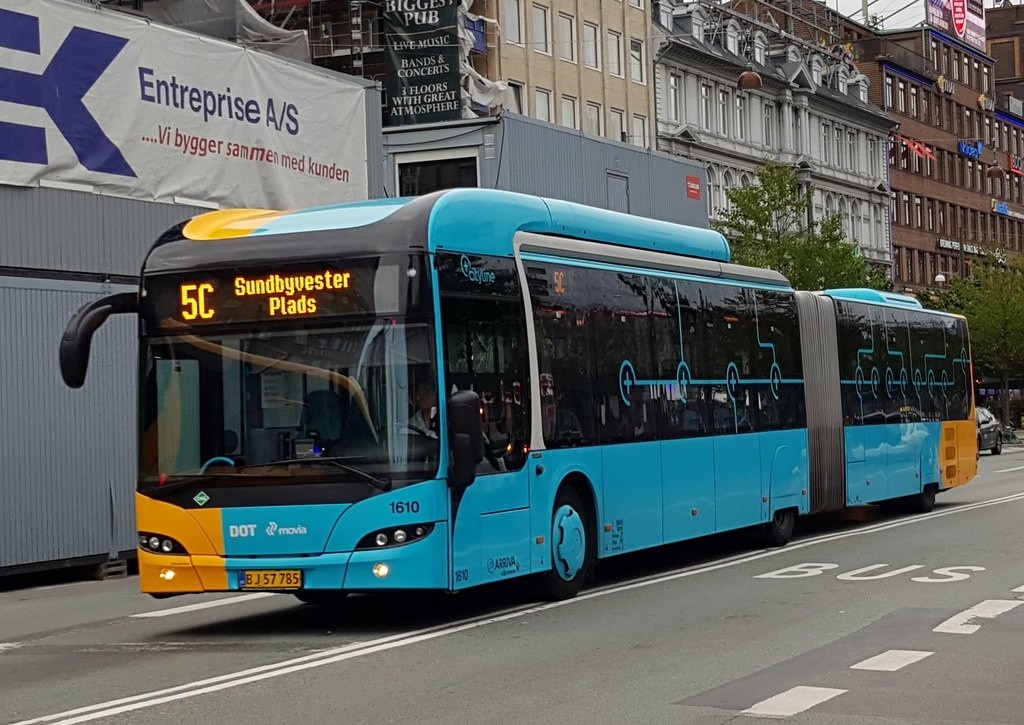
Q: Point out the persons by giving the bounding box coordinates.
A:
[398,380,437,439]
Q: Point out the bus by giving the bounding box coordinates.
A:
[56,187,980,607]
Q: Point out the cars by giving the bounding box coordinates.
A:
[976,405,1003,455]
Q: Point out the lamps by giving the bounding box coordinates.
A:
[675,63,763,90]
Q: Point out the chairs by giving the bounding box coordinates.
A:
[302,389,344,440]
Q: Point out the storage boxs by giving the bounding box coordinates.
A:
[464,17,486,55]
